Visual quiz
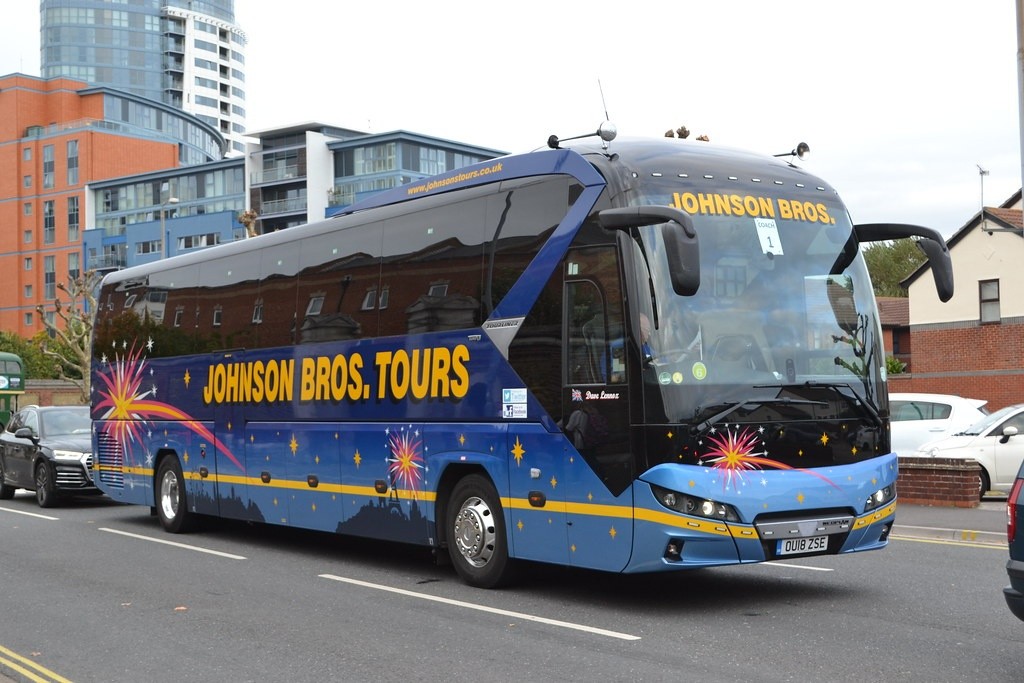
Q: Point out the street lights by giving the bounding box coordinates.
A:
[160,197,180,260]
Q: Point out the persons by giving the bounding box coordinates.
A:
[602,312,667,381]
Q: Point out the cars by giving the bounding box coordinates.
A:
[920,405,1024,499]
[1003,458,1024,621]
[888,393,989,453]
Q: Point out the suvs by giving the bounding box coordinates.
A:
[0,403,105,508]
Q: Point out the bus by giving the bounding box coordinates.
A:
[0,351,28,433]
[89,135,955,592]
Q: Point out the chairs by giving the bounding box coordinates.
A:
[581,314,621,384]
